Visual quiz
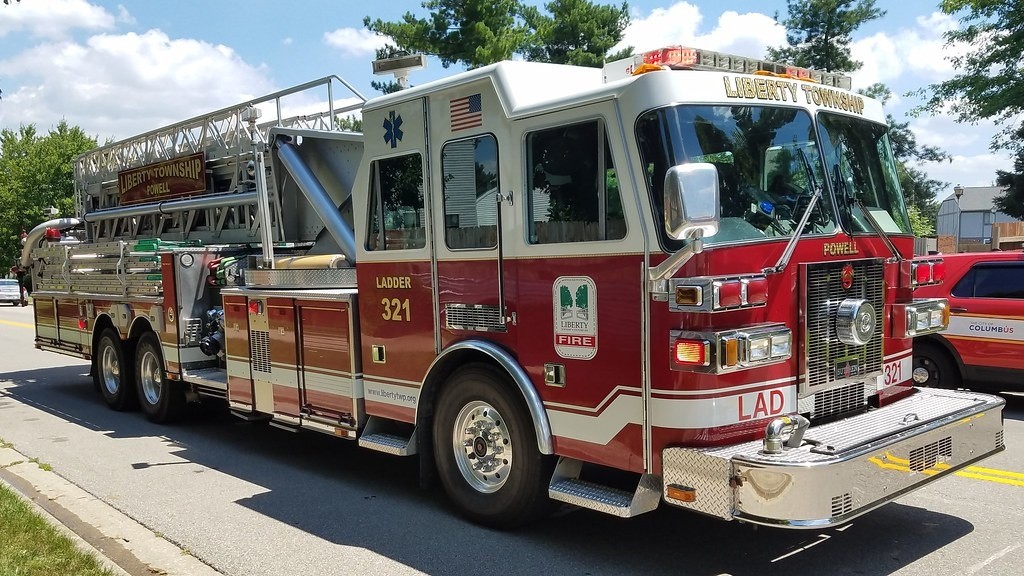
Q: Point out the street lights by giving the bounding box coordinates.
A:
[990,205,997,224]
[954,183,964,253]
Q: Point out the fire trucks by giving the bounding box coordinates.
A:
[24,46,1007,530]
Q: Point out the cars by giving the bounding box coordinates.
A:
[0,279,28,306]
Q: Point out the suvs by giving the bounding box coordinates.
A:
[911,251,1024,398]
